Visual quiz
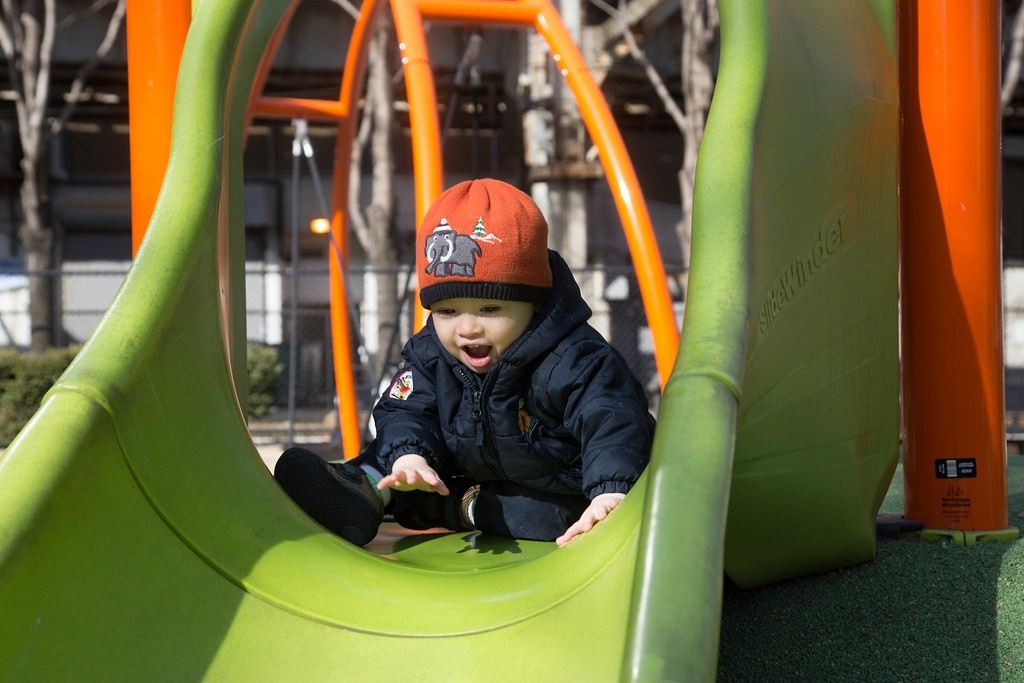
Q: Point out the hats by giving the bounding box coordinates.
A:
[415,178,555,304]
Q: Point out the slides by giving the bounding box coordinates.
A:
[0,0,773,682]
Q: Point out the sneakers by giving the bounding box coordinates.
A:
[276,446,383,546]
[391,474,482,533]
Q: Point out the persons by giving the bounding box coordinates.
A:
[272,177,656,546]
